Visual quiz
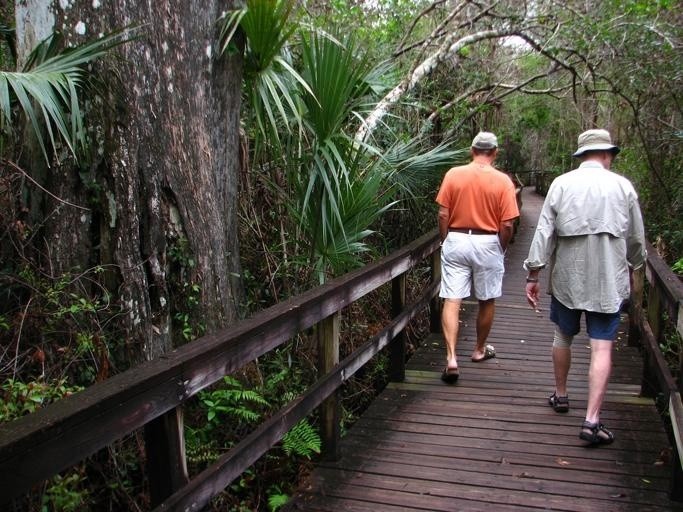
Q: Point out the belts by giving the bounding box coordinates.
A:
[449,227,497,235]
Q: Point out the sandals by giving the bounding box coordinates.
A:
[579,421,614,445]
[548,391,569,413]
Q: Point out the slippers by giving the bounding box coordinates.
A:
[441,368,459,382]
[472,344,496,362]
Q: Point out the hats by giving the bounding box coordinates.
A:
[471,131,499,149]
[572,129,620,157]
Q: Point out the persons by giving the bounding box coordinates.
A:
[434,132,521,379]
[526,129,648,445]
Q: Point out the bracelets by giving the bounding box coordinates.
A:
[526,276,539,283]
